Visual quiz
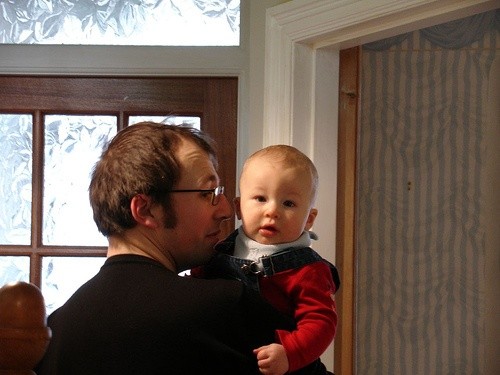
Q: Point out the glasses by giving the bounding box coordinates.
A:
[164,186,228,205]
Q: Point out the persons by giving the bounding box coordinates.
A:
[184,143,338,373]
[37,120,334,374]
